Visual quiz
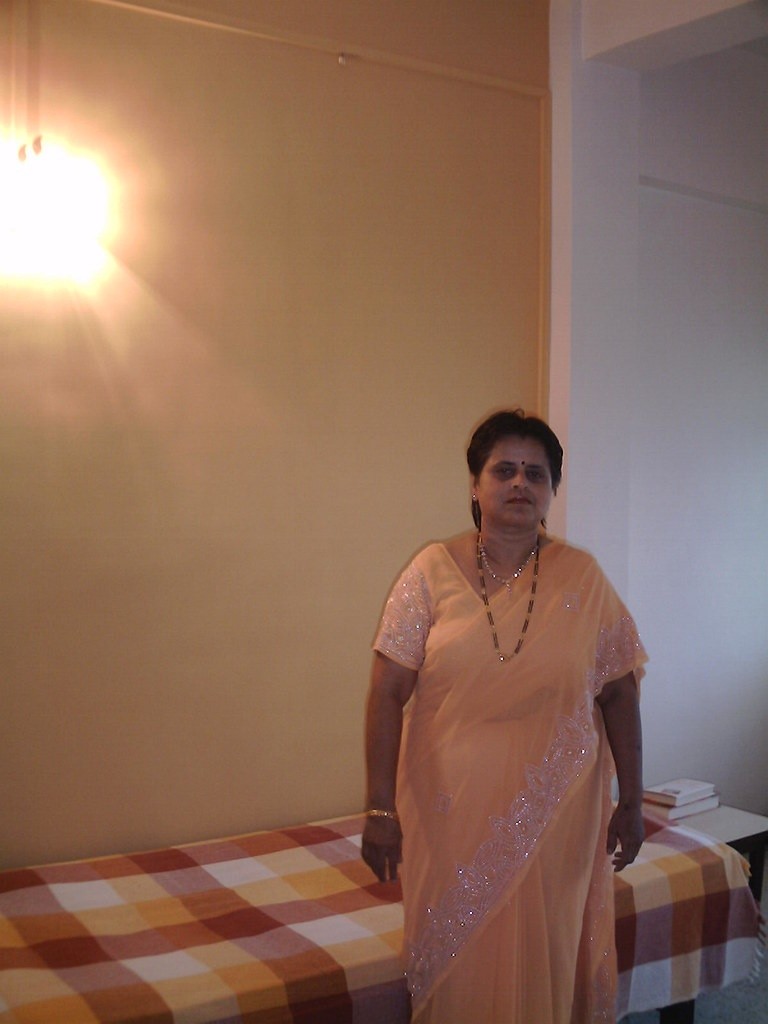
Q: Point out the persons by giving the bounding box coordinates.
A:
[361,405,649,1024]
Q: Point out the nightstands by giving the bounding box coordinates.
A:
[671,802,768,903]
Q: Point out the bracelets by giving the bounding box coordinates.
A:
[362,808,400,821]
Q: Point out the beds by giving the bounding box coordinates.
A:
[0,793,762,1023]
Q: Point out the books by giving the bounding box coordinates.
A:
[641,778,720,821]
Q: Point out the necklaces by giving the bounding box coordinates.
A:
[475,528,542,663]
